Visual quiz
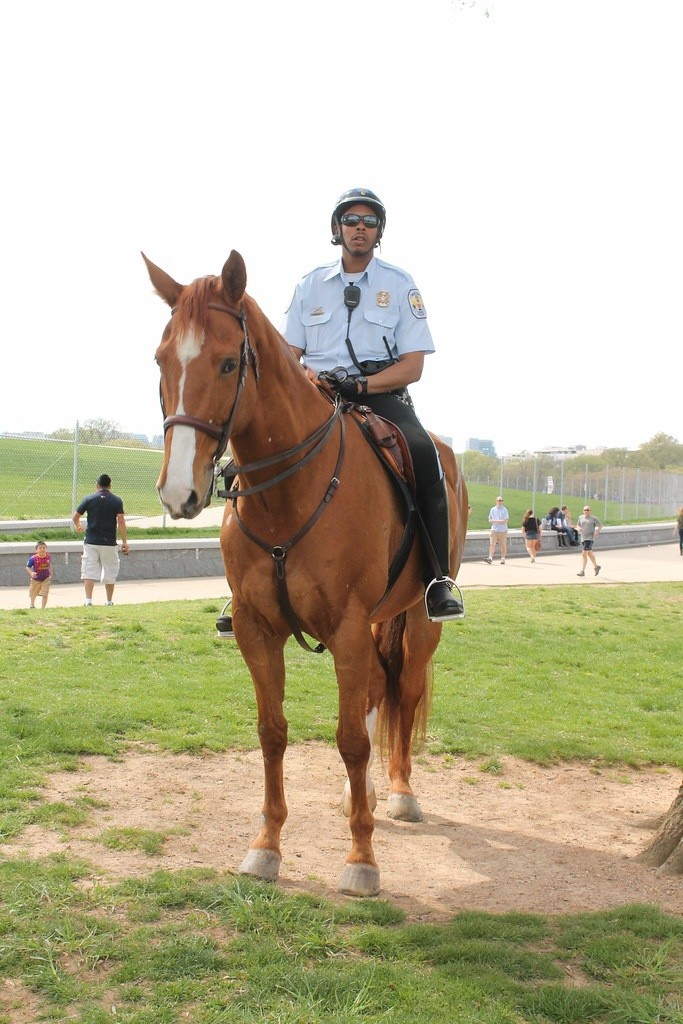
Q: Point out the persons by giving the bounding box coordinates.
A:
[72,475,129,606]
[216,188,462,633]
[545,506,580,548]
[673,508,683,556]
[484,496,510,564]
[576,506,602,577]
[26,542,53,609]
[522,510,541,563]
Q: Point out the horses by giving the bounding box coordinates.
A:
[140,250,469,897]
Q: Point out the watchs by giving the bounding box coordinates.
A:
[357,377,368,395]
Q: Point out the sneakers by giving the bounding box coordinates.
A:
[594,565,601,576]
[576,571,585,576]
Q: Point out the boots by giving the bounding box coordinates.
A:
[407,472,464,617]
[215,614,233,631]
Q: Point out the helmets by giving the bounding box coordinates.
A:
[330,189,385,233]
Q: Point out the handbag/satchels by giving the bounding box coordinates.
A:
[535,539,541,551]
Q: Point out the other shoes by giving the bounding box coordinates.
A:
[104,601,113,606]
[531,557,536,562]
[483,558,492,564]
[30,606,35,608]
[500,558,505,564]
[84,603,93,607]
[563,544,567,546]
[558,545,562,548]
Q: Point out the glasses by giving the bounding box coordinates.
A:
[340,214,381,229]
[583,509,590,511]
[497,500,503,502]
